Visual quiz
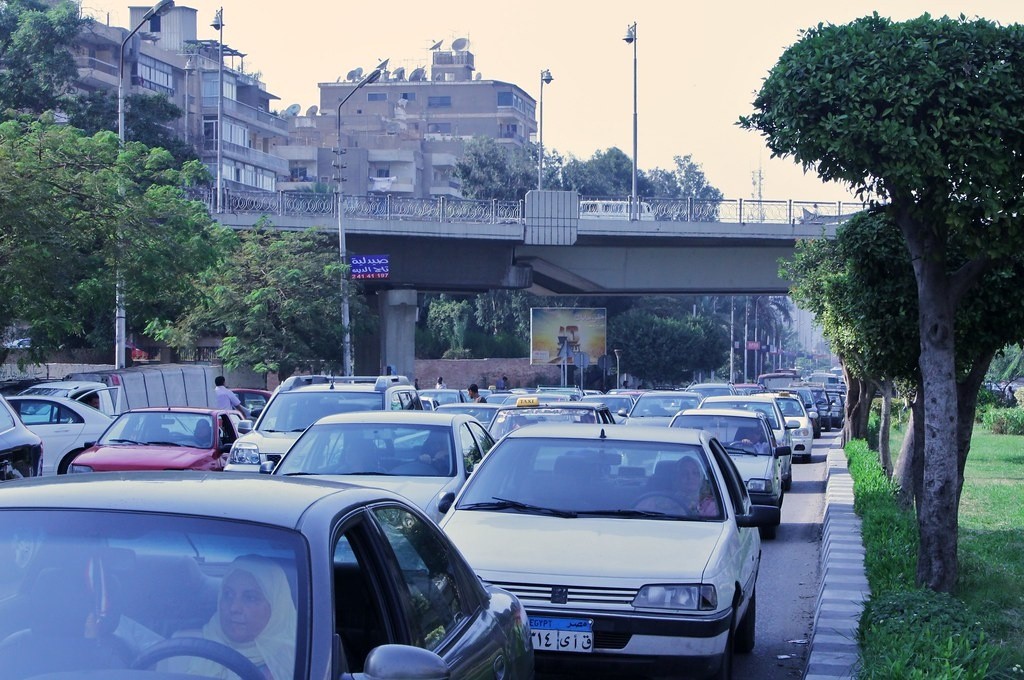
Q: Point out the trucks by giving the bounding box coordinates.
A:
[15,357,225,436]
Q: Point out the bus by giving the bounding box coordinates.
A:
[578,200,656,222]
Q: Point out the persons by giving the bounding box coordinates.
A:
[418,432,449,465]
[785,402,792,414]
[194,419,222,446]
[742,427,762,446]
[468,384,488,403]
[435,377,446,389]
[503,377,509,389]
[817,392,826,402]
[1007,386,1017,403]
[215,376,246,416]
[415,378,418,389]
[672,455,716,516]
[158,553,297,680]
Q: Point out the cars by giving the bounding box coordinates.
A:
[374,367,850,440]
[66,406,247,472]
[225,387,274,422]
[7,337,33,349]
[271,409,497,570]
[652,408,792,541]
[694,395,801,492]
[3,394,129,480]
[0,470,536,680]
[413,421,781,679]
[0,392,45,484]
[743,390,820,464]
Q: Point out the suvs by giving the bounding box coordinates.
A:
[223,374,426,476]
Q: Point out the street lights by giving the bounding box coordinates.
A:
[113,0,177,371]
[538,68,554,190]
[614,349,623,389]
[209,7,226,212]
[335,67,384,377]
[623,20,638,221]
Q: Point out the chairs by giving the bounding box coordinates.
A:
[548,456,596,504]
[333,439,386,473]
[651,459,677,490]
